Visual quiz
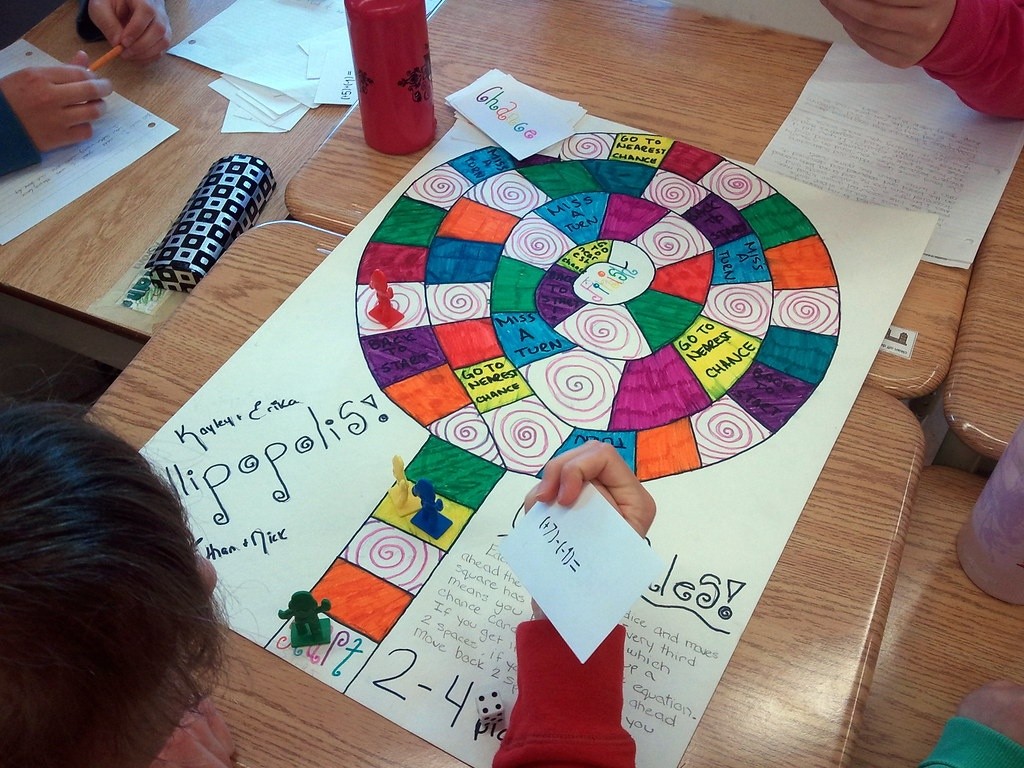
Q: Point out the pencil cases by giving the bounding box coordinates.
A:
[143,153,279,292]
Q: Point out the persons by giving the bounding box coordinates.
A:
[0,0,171,177]
[1,402,657,768]
[918,679,1024,768]
[820,0,1024,120]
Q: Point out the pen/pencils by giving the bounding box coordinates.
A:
[83,43,125,73]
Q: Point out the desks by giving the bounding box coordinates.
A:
[0,0,1024,768]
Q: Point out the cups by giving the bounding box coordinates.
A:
[344,0,435,155]
